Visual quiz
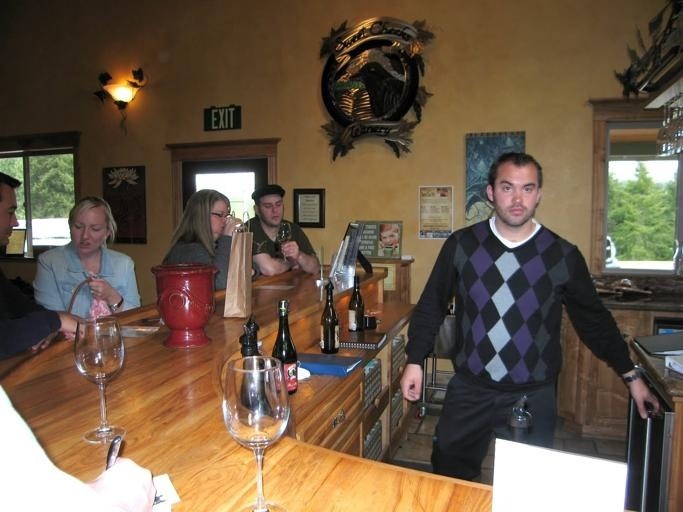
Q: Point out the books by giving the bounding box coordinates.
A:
[665,354,683,375]
[313,330,386,351]
[634,331,683,356]
[327,223,362,278]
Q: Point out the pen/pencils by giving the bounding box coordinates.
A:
[106,436,123,470]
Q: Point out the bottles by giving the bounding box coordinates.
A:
[270,299,300,393]
[318,276,341,354]
[239,320,258,356]
[345,274,364,334]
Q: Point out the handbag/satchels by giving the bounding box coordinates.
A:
[224,211,253,319]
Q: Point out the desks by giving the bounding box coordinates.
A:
[355,256,415,305]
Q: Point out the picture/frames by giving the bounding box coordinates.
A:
[292,188,327,229]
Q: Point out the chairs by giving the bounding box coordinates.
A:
[416,315,456,419]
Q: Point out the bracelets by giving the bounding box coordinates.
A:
[622,371,641,384]
[110,296,123,311]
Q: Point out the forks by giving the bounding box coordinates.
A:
[220,355,292,511]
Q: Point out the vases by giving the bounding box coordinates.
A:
[148,262,222,349]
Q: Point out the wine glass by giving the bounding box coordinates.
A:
[275,221,295,266]
[228,218,267,254]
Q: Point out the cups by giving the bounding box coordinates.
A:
[363,314,376,329]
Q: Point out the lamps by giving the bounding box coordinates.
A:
[91,66,151,139]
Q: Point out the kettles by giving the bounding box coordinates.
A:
[72,317,127,446]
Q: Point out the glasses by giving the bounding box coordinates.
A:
[211,212,232,219]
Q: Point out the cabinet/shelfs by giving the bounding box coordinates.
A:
[574,305,656,441]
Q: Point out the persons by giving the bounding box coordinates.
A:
[399,152,662,482]
[0,378,157,510]
[238,184,321,276]
[31,195,141,320]
[0,171,84,358]
[378,224,398,247]
[161,189,259,292]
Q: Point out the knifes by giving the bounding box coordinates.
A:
[103,432,125,469]
